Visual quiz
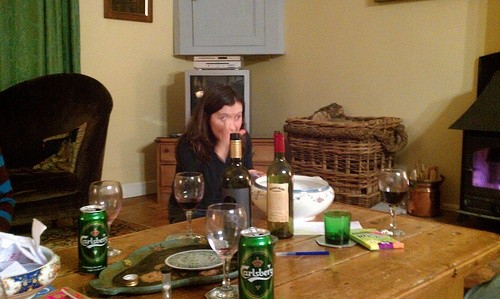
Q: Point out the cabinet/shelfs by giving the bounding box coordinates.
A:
[154,137,177,206]
[173,0,285,56]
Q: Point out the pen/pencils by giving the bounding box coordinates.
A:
[276,251,329,256]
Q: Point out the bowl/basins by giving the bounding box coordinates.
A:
[251,175,335,221]
[0,245,61,299]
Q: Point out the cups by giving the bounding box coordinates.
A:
[323,210,351,246]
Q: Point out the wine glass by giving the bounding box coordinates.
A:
[377,168,410,236]
[205,202,248,299]
[173,172,205,235]
[89,180,122,255]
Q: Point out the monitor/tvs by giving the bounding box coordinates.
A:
[184,69,250,135]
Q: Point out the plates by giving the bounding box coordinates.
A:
[88,235,238,294]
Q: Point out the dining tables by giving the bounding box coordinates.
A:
[0,201,500,299]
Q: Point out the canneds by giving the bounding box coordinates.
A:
[78,204,108,275]
[237,226,274,299]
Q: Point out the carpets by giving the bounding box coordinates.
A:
[5,216,150,250]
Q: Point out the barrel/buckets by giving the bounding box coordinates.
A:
[409,165,445,217]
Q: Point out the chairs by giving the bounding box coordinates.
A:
[0,72,114,234]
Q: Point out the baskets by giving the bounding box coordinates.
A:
[283,113,408,209]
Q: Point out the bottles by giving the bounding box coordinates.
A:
[221,132,252,237]
[266,131,294,238]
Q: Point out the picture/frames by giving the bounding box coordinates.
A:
[104,0,153,23]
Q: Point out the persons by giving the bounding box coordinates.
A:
[168,84,253,223]
